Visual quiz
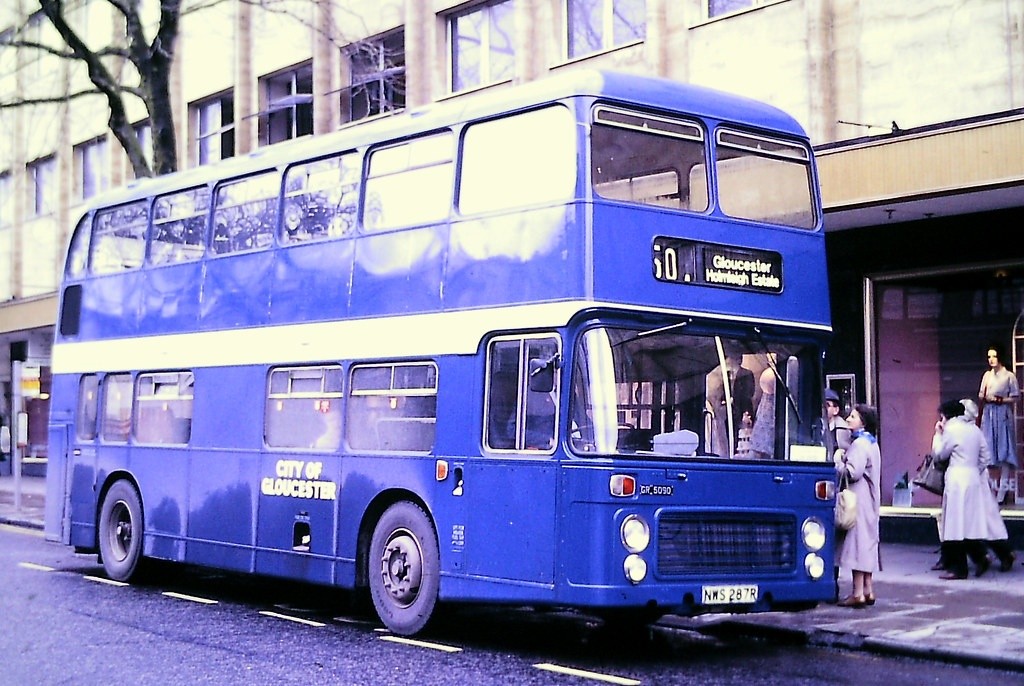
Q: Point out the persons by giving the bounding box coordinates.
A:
[720,353,755,454]
[752,367,776,458]
[929,399,1015,580]
[506,368,590,448]
[822,388,852,451]
[834,405,881,608]
[979,348,1021,506]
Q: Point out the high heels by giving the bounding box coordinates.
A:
[865,594,874,606]
[838,595,866,609]
[998,489,1009,505]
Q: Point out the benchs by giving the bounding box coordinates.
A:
[373,415,436,452]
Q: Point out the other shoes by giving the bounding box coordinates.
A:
[975,556,991,576]
[931,560,946,570]
[1001,552,1015,571]
[939,572,967,579]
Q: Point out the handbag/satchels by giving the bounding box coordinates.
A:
[913,455,947,496]
[835,469,857,530]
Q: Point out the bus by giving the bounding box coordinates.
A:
[43,74,841,640]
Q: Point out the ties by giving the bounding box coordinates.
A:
[731,371,734,396]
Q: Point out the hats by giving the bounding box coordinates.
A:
[824,388,840,403]
